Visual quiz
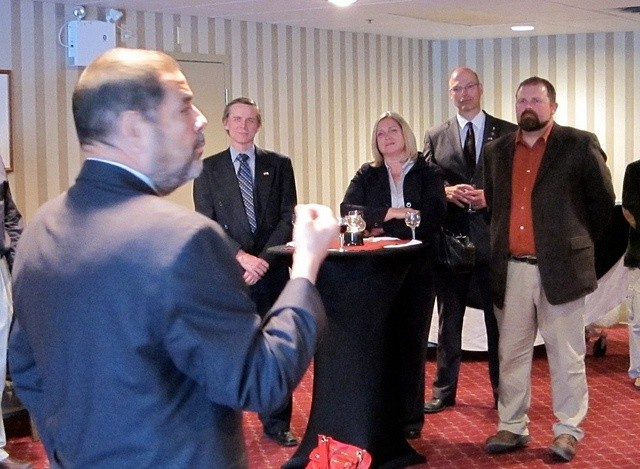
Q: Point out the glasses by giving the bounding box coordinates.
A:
[449,82,478,93]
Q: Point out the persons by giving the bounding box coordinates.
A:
[193,99,299,447]
[0,157,32,465]
[7,47,336,469]
[423,68,520,413]
[483,78,613,460]
[340,111,437,437]
[622,161,640,388]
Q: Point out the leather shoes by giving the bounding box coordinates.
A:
[485,428,530,454]
[423,396,457,413]
[265,429,297,445]
[548,433,577,460]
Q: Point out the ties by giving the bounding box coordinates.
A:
[235,153,257,233]
[463,121,476,178]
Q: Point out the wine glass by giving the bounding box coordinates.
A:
[405,208,422,245]
[465,183,476,213]
[348,210,364,229]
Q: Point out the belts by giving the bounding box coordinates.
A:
[507,255,539,265]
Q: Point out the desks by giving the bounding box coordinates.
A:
[266,237,438,469]
